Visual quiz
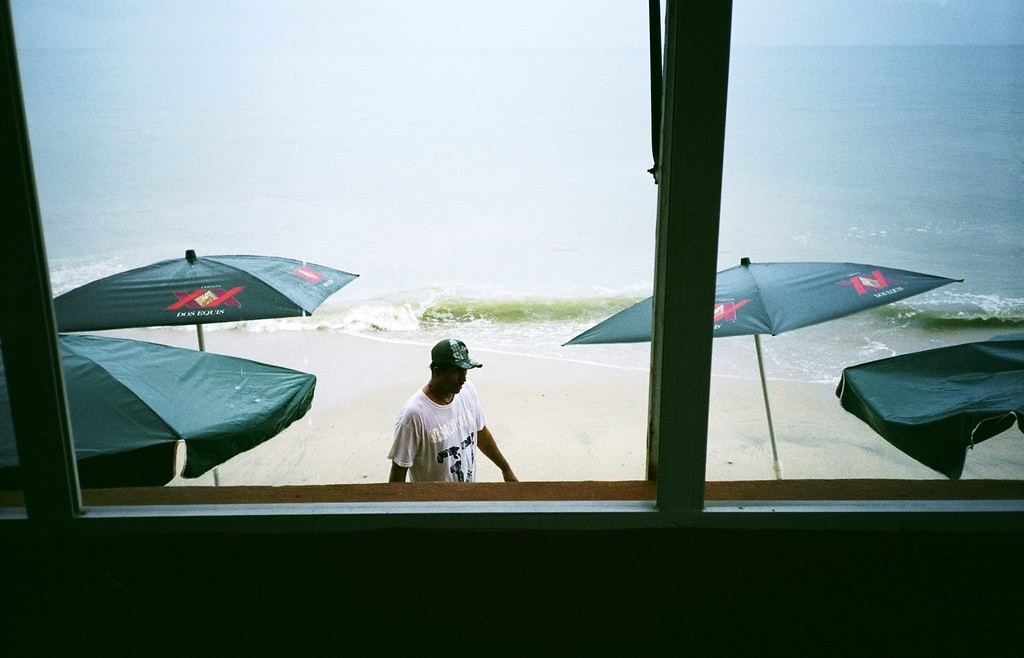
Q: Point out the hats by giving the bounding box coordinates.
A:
[431,339,484,369]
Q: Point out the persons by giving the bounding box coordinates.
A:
[388,339,520,482]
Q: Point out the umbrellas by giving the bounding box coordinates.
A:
[837,331,1024,480]
[54,250,361,485]
[56,334,316,487]
[562,260,963,480]
